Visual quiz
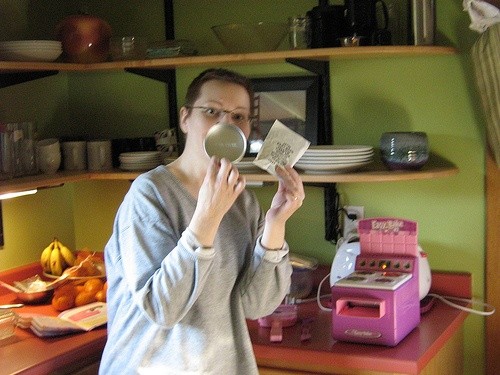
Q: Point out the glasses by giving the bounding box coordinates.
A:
[188,105,254,126]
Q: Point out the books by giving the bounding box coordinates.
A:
[31,301,107,337]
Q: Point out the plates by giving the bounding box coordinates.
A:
[118,150,180,170]
[44,264,106,283]
[0,39,62,62]
[230,143,375,178]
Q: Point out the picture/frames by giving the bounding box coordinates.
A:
[245,74,321,158]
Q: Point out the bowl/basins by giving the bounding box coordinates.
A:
[287,268,314,298]
[258,305,297,327]
[14,280,55,302]
[108,37,148,60]
[211,22,289,55]
[149,39,198,59]
[0,308,17,341]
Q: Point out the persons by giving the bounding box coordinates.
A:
[98,68,305,375]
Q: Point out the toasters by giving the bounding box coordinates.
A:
[329,233,432,306]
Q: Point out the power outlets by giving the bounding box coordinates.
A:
[343,206,364,235]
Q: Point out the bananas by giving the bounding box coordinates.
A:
[41,237,76,276]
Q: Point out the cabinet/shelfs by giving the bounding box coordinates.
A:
[0,45,462,244]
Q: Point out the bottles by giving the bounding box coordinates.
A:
[56,15,114,63]
[405,0,436,47]
[306,0,343,49]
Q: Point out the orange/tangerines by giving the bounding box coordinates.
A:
[52,278,107,310]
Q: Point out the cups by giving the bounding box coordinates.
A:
[377,30,391,46]
[343,0,389,47]
[379,131,430,171]
[87,139,112,170]
[63,141,87,171]
[0,122,20,180]
[39,138,61,150]
[340,36,361,48]
[288,16,312,50]
[19,119,39,176]
[39,150,61,174]
